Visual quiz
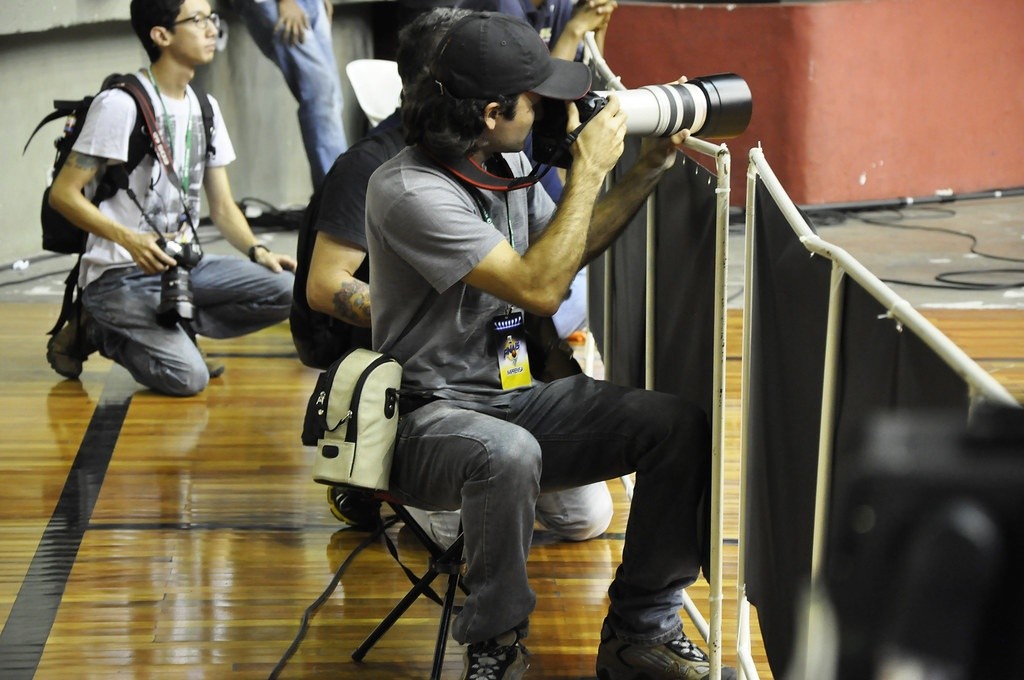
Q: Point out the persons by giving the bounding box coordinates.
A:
[46,0,297,398]
[237,0,346,194]
[286,0,619,543]
[365,12,736,680]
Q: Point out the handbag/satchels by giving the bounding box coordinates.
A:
[299,348,403,489]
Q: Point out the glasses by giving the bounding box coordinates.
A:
[173,12,221,29]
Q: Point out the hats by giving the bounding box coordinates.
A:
[430,10,592,100]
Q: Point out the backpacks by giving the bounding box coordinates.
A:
[24,74,215,253]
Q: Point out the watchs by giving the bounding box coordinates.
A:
[247,244,269,263]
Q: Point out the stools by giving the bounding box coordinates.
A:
[351,491,471,680]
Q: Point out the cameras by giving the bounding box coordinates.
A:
[532,73,754,168]
[156,238,204,324]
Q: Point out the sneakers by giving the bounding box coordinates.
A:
[461,621,533,680]
[47,316,98,379]
[596,627,737,680]
[184,319,225,378]
[326,481,382,528]
